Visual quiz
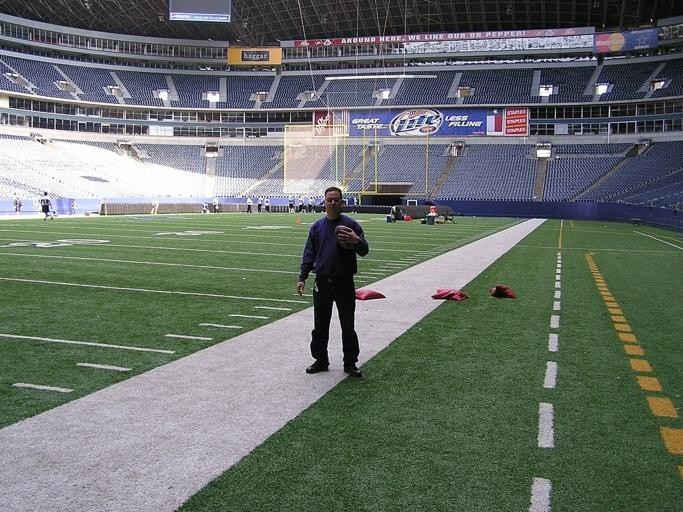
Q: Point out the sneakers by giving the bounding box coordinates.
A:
[306,359,328,373]
[344,362,362,377]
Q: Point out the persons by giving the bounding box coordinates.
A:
[151,199,158,214]
[244,195,317,213]
[201,201,209,214]
[41,191,54,221]
[297,187,369,378]
[213,196,219,213]
[71,201,77,214]
[14,197,21,214]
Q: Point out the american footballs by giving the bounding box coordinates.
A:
[334,225,356,250]
